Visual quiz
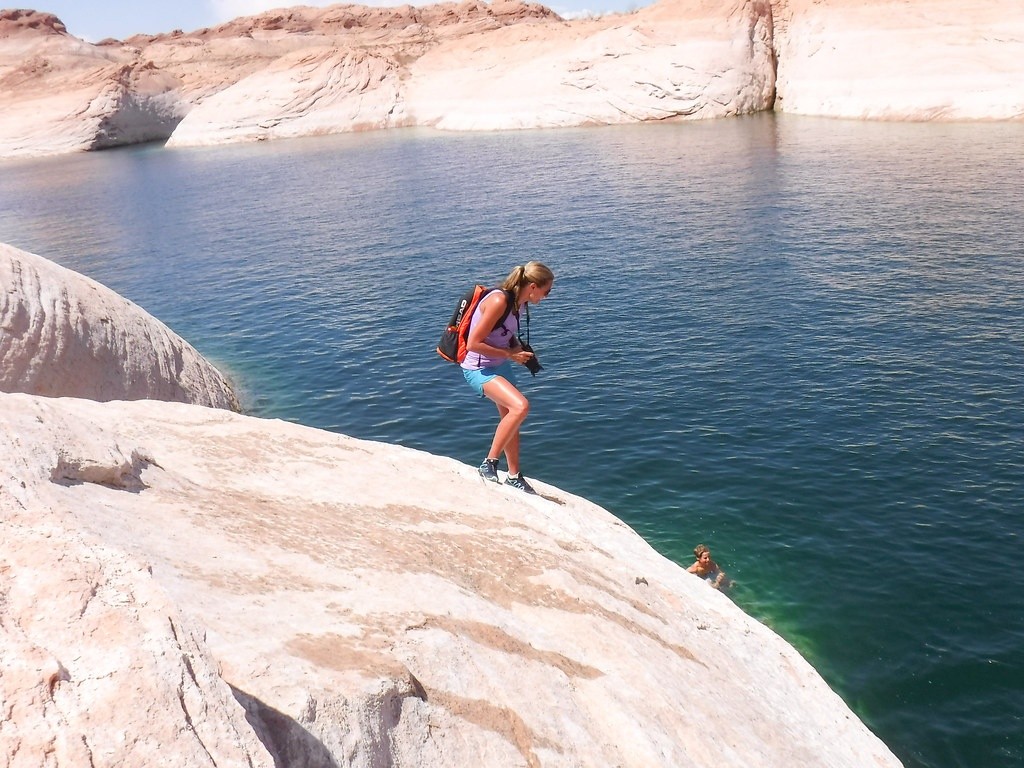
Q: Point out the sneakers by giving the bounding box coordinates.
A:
[477,457,500,482]
[503,470,537,494]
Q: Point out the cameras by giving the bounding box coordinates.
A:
[521,345,544,378]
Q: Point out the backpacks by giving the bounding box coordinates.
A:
[436,283,520,365]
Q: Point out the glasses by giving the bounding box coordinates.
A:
[544,288,551,297]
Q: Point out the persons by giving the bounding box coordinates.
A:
[685,544,732,589]
[460,261,555,494]
